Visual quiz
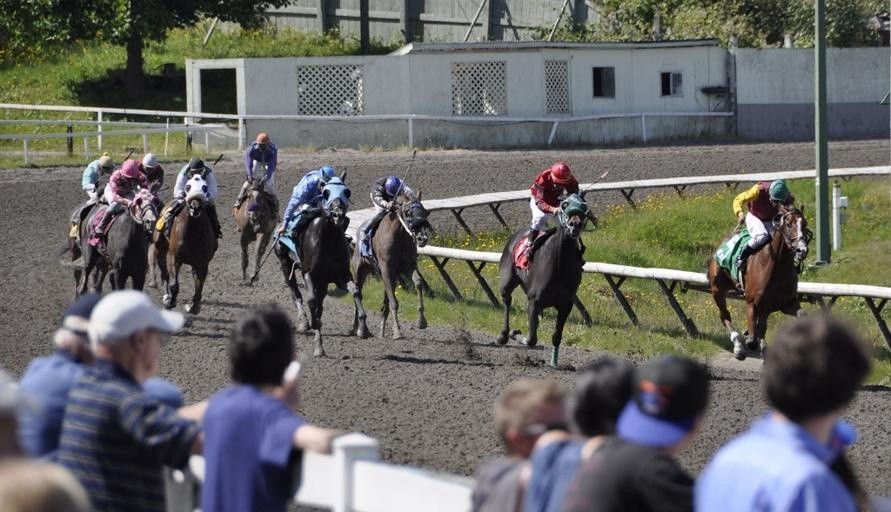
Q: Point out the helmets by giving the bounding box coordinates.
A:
[385,177,403,196]
[188,157,205,172]
[317,165,335,183]
[770,180,789,200]
[257,132,271,144]
[551,162,573,185]
[100,151,158,178]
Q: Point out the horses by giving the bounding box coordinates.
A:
[147,179,175,287]
[234,174,279,280]
[154,166,218,315]
[67,162,115,299]
[274,166,369,358]
[75,177,157,295]
[707,202,809,361]
[494,186,587,369]
[350,189,428,341]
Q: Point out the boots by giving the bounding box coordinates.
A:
[233,188,248,208]
[525,228,539,257]
[271,193,281,222]
[735,243,755,271]
[72,203,88,223]
[278,215,302,247]
[360,215,382,240]
[94,211,111,234]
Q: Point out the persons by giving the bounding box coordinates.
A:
[80,154,216,238]
[278,165,336,239]
[1,280,352,511]
[521,159,581,246]
[360,177,415,241]
[732,179,802,269]
[466,310,876,509]
[233,132,281,222]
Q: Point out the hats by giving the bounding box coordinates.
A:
[57,288,187,341]
[612,355,708,446]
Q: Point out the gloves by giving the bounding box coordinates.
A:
[259,178,264,186]
[247,175,253,183]
